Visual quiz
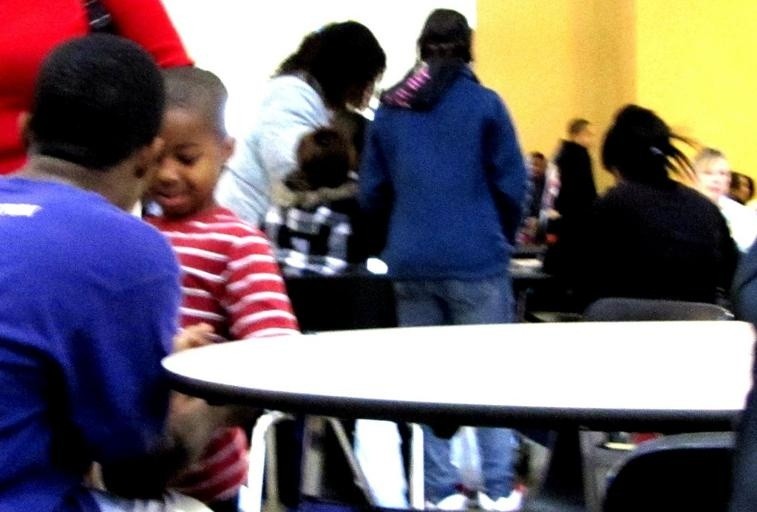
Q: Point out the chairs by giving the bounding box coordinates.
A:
[243,275,399,506]
[594,431,737,512]
[575,298,736,512]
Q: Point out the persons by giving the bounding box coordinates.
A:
[1,0,757,510]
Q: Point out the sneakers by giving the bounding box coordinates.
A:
[425,493,468,512]
[477,490,524,512]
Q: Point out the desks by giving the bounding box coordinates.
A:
[503,253,556,319]
[157,320,757,512]
